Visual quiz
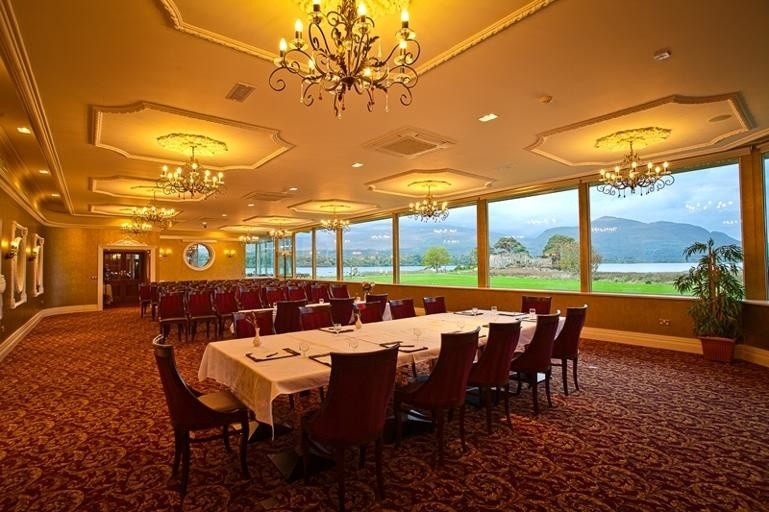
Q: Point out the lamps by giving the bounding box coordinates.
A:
[594,126,675,198]
[408,179,452,224]
[318,204,351,234]
[267,0,420,121]
[156,134,228,198]
[121,200,177,234]
[270,228,293,239]
[237,231,260,245]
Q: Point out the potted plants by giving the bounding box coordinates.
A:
[673,237,745,363]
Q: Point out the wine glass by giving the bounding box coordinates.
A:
[319,299,324,304]
[414,327,423,341]
[530,308,536,320]
[457,320,465,330]
[273,302,277,310]
[350,339,360,352]
[472,307,479,320]
[491,305,497,318]
[299,343,310,359]
[334,324,342,336]
